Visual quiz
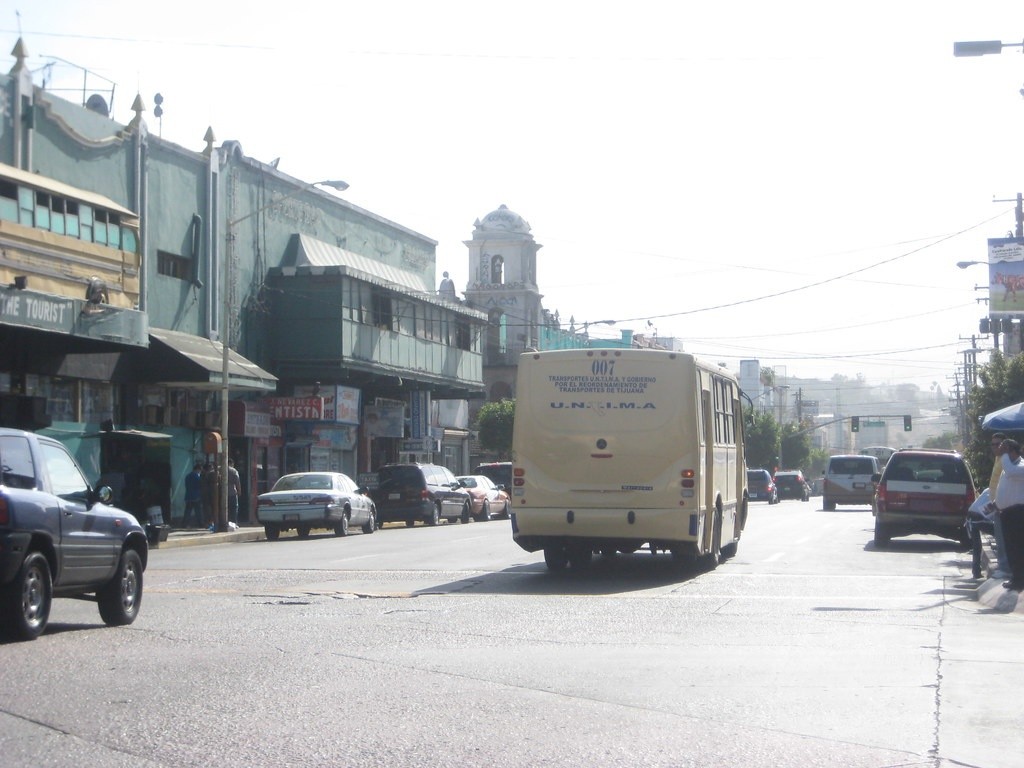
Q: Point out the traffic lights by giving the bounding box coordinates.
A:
[851,416,859,432]
[905,416,912,432]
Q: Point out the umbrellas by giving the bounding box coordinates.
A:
[982,402,1024,431]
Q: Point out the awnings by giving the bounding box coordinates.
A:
[0,283,150,351]
[135,327,279,393]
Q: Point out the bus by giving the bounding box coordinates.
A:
[507,350,755,571]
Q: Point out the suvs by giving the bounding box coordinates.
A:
[871,448,977,547]
[772,470,811,502]
[0,427,152,643]
[821,456,883,510]
[370,465,470,526]
[746,468,779,504]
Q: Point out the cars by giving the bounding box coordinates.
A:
[256,471,377,540]
[455,475,512,523]
[476,462,512,494]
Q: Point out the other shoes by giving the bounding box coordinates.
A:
[1002,580,1024,590]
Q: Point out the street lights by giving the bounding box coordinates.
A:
[546,320,614,348]
[749,385,789,408]
[217,177,350,532]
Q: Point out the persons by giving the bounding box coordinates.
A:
[989,433,1013,578]
[181,457,242,530]
[983,439,1024,590]
[967,487,996,579]
